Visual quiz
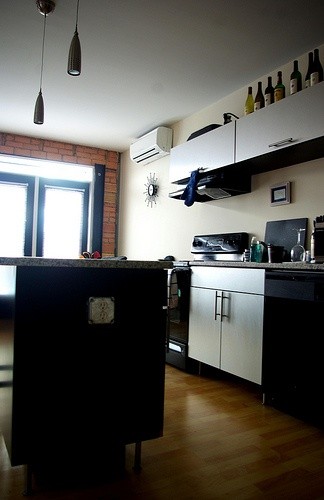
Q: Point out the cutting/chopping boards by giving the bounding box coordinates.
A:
[264,218,308,253]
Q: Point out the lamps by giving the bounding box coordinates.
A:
[67,0,82,76]
[34,0,56,125]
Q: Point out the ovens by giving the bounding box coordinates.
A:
[165,266,197,372]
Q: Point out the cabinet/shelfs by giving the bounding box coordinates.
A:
[169,82,324,184]
[189,266,266,405]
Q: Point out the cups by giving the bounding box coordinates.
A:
[267,245,287,263]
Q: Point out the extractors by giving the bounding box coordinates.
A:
[169,171,251,202]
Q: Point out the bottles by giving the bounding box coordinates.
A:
[309,49,324,87]
[264,77,275,107]
[290,61,302,96]
[249,236,263,263]
[274,71,285,103]
[245,86,255,116]
[305,51,314,88]
[253,82,264,112]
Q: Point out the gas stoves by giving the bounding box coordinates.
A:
[170,233,247,266]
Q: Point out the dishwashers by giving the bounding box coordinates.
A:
[261,269,324,429]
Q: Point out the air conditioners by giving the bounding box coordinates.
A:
[129,127,172,166]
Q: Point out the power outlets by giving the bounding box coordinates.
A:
[88,297,115,324]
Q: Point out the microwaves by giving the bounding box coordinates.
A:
[310,222,324,263]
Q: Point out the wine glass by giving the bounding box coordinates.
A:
[290,228,306,262]
